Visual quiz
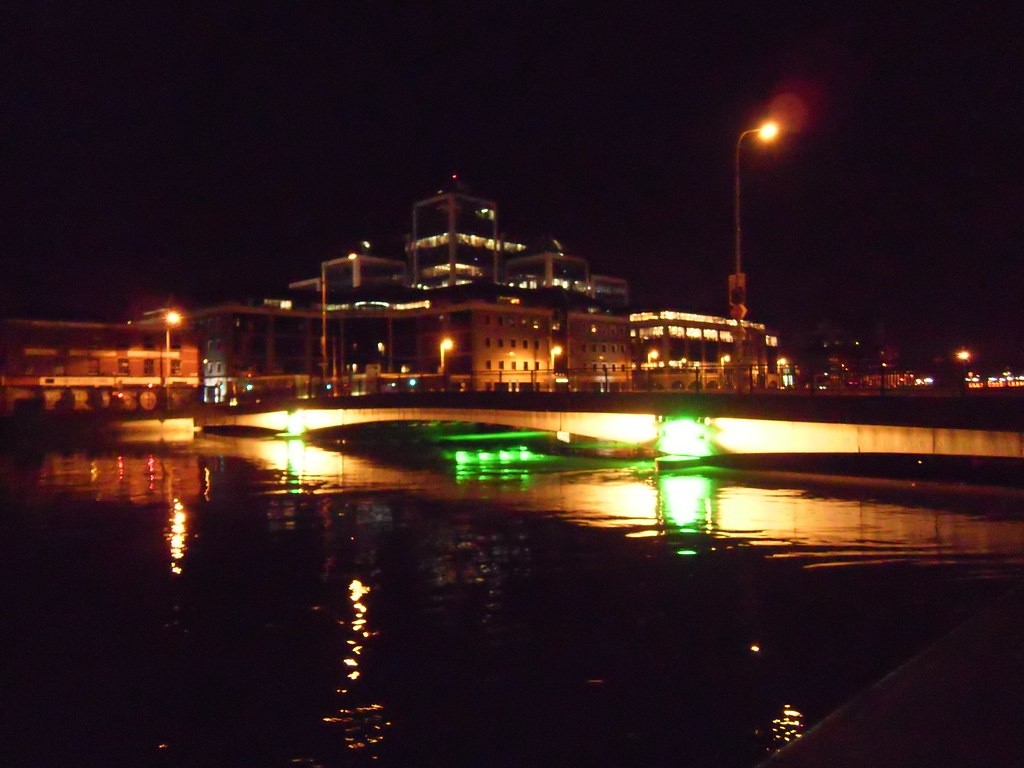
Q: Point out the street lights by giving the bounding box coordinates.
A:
[729,121,780,389]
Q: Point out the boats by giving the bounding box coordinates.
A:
[194,376,622,413]
